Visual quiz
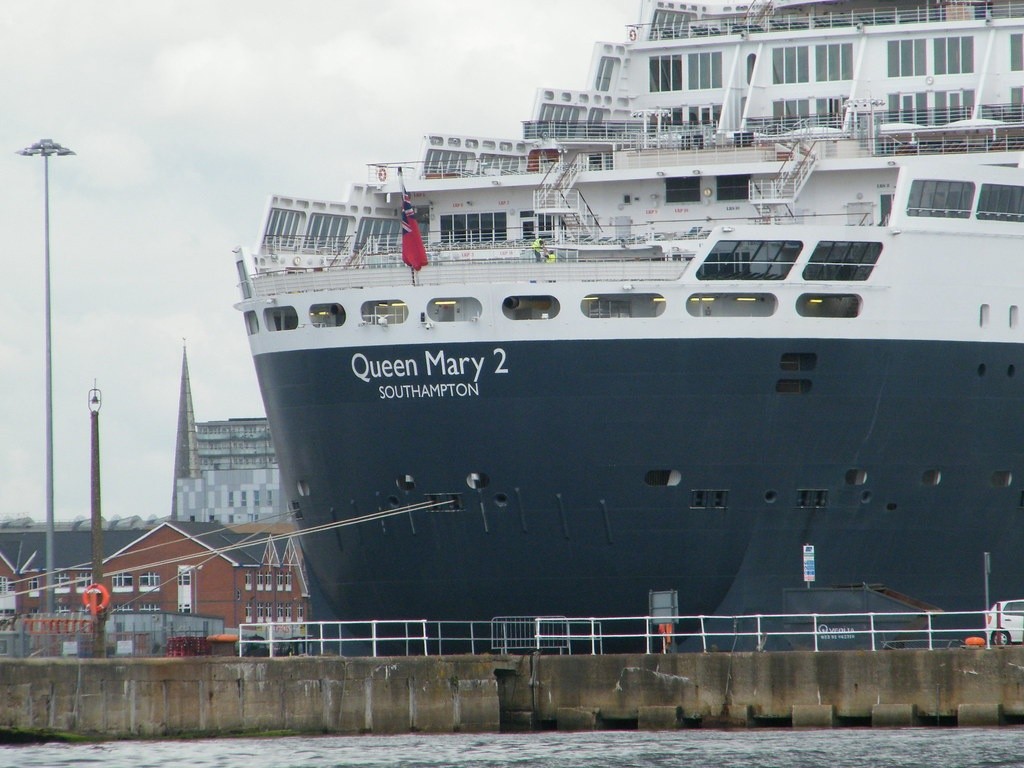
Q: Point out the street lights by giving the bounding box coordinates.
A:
[16,139,77,616]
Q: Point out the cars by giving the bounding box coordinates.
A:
[988,599,1024,645]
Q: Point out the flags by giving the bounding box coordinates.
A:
[401,185,428,272]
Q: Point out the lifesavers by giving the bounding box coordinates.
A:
[82,584,111,613]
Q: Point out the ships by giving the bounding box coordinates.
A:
[227,1,1024,656]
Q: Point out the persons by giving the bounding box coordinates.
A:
[543,246,556,263]
[532,238,544,263]
